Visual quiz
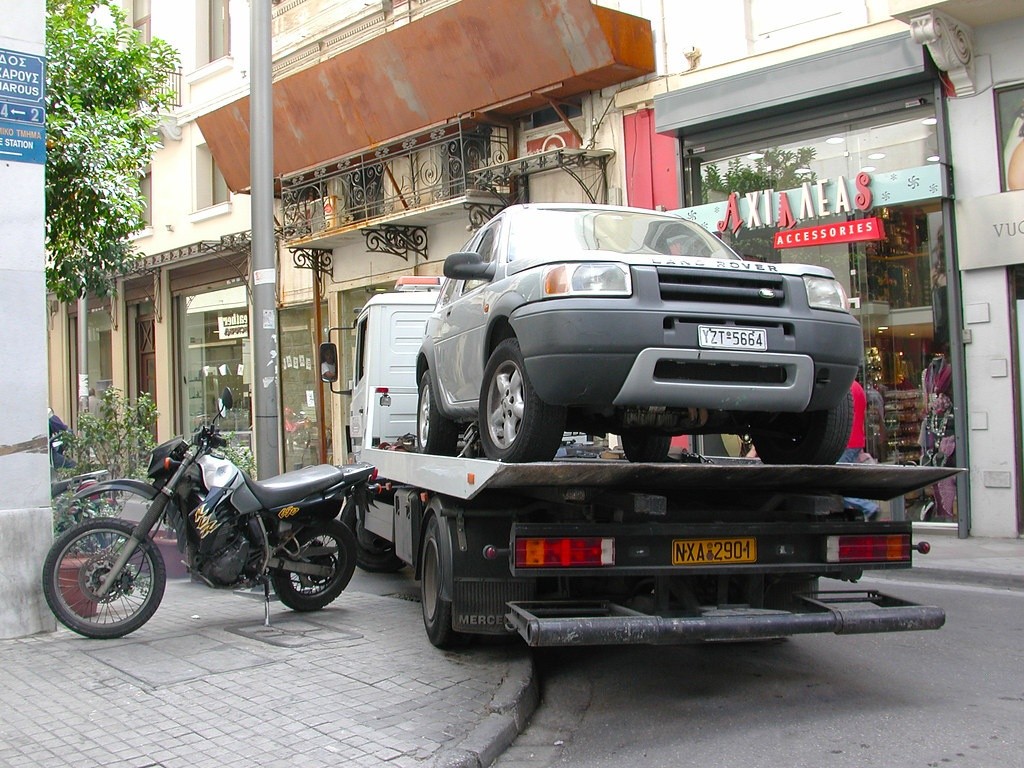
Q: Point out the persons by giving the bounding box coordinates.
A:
[837,380,870,462]
[46,407,70,456]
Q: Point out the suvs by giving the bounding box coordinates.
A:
[415,201,865,467]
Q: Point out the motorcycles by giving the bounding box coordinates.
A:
[49,433,109,551]
[41,386,381,641]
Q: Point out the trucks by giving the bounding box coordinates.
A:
[318,275,969,650]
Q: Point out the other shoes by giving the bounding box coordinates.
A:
[867,511,878,523]
